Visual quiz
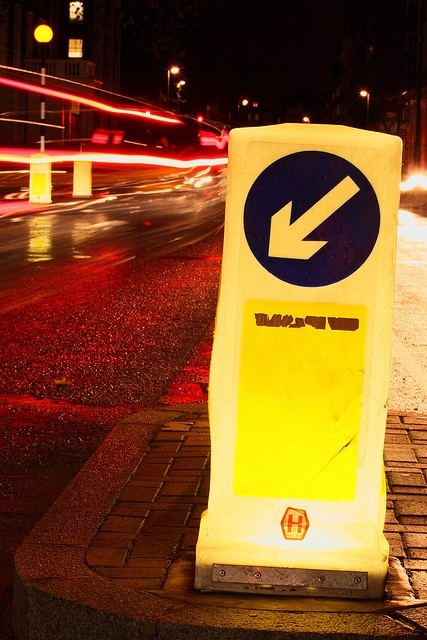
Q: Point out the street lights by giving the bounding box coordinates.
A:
[360,90,370,128]
[167,67,179,106]
[238,96,248,124]
[32,17,53,152]
